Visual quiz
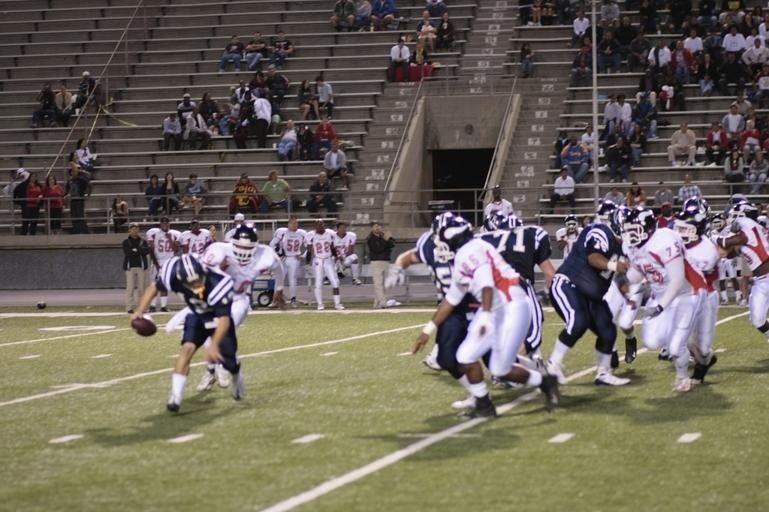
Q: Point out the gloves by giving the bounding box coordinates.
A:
[383,265,405,289]
[635,304,663,320]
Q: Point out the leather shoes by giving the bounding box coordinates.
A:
[128,310,134,313]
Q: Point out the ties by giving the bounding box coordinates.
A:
[399,48,402,58]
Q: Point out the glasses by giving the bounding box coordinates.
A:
[320,176,327,178]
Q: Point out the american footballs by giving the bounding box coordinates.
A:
[131,317,157,337]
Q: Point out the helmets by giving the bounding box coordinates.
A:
[170,254,206,296]
[232,225,258,265]
[597,201,619,222]
[679,196,711,235]
[710,214,725,231]
[298,249,309,260]
[725,193,759,233]
[434,216,473,264]
[564,215,578,233]
[609,206,632,240]
[430,212,453,247]
[498,215,523,230]
[623,208,656,248]
[275,244,285,257]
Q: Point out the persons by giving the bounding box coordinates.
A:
[731,90,751,116]
[673,209,721,386]
[746,27,765,49]
[745,107,766,142]
[644,38,671,90]
[130,254,245,413]
[547,199,638,386]
[741,38,769,75]
[712,200,769,343]
[366,222,395,309]
[596,0,769,38]
[228,172,260,220]
[75,138,96,180]
[412,215,559,422]
[368,0,394,31]
[598,31,621,74]
[549,168,575,214]
[752,64,769,109]
[416,10,437,53]
[323,222,362,285]
[175,217,217,255]
[749,151,769,194]
[671,40,693,85]
[51,83,73,127]
[599,93,617,141]
[69,152,90,197]
[559,136,589,183]
[165,222,283,393]
[262,170,293,219]
[739,120,762,166]
[268,31,294,70]
[268,215,313,309]
[313,75,334,120]
[353,0,372,32]
[622,205,708,392]
[206,108,224,136]
[530,0,542,26]
[569,34,600,77]
[306,171,337,218]
[390,39,411,82]
[678,173,701,206]
[3,167,25,195]
[266,64,289,104]
[224,212,244,243]
[297,124,315,161]
[634,92,656,143]
[655,202,673,229]
[553,130,571,169]
[558,0,592,25]
[703,121,728,165]
[652,181,674,208]
[602,206,648,373]
[470,216,556,389]
[629,124,646,167]
[59,162,92,234]
[625,182,647,207]
[14,172,44,236]
[38,175,65,234]
[236,79,249,104]
[519,0,532,26]
[627,32,652,73]
[297,79,320,121]
[602,187,624,206]
[182,172,208,214]
[160,172,185,216]
[304,219,345,311]
[567,9,589,49]
[330,0,356,32]
[721,106,745,152]
[255,92,272,149]
[667,121,697,166]
[581,126,594,169]
[418,21,437,39]
[112,197,129,233]
[519,42,535,77]
[162,114,182,151]
[245,32,267,71]
[436,12,453,53]
[717,52,746,96]
[738,254,752,309]
[184,104,212,150]
[639,68,658,92]
[657,195,740,362]
[123,222,150,314]
[226,97,241,136]
[32,81,57,128]
[177,93,197,131]
[383,211,549,408]
[324,138,349,188]
[314,115,337,160]
[724,149,745,195]
[218,34,244,72]
[711,213,742,305]
[250,71,265,91]
[426,0,447,17]
[702,29,723,48]
[541,0,556,26]
[609,136,630,183]
[75,71,102,116]
[146,217,182,313]
[721,26,745,54]
[608,93,632,138]
[606,124,627,145]
[556,215,584,260]
[483,185,514,220]
[684,29,704,55]
[696,53,717,97]
[199,92,218,122]
[281,120,295,161]
[410,43,428,80]
[143,175,163,222]
[239,91,255,112]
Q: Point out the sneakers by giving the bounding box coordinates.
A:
[622,179,627,183]
[161,306,171,312]
[457,404,496,419]
[218,367,231,388]
[721,298,729,305]
[750,190,758,194]
[736,299,748,307]
[625,337,637,363]
[290,302,298,308]
[609,179,616,183]
[247,306,252,312]
[668,351,696,367]
[423,353,443,370]
[611,350,619,368]
[335,304,344,310]
[545,374,559,412]
[452,396,478,409]
[166,394,182,415]
[532,354,549,376]
[149,306,156,312]
[658,346,671,362]
[673,377,691,392]
[652,135,659,139]
[197,375,216,392]
[269,303,278,308]
[233,373,244,400]
[595,369,631,386]
[545,360,568,385]
[317,304,324,310]
[692,356,717,383]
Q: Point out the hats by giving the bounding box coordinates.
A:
[16,168,27,179]
[184,93,191,98]
[192,219,200,225]
[190,174,198,178]
[83,71,90,76]
[253,87,266,98]
[240,173,248,179]
[235,213,245,220]
[129,222,139,229]
[161,217,169,224]
[371,220,379,226]
